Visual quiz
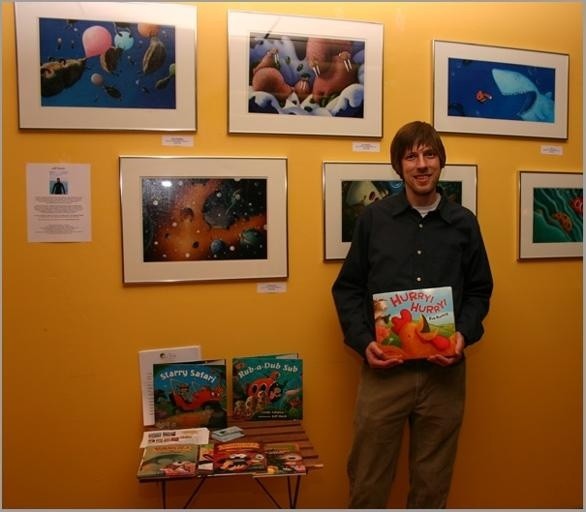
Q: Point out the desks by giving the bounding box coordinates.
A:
[138,418,325,509]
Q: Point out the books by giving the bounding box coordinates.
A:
[136,345,307,478]
[373,286,457,361]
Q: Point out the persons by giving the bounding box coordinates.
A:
[332,121,493,509]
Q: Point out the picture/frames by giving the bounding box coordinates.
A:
[10,3,584,286]
